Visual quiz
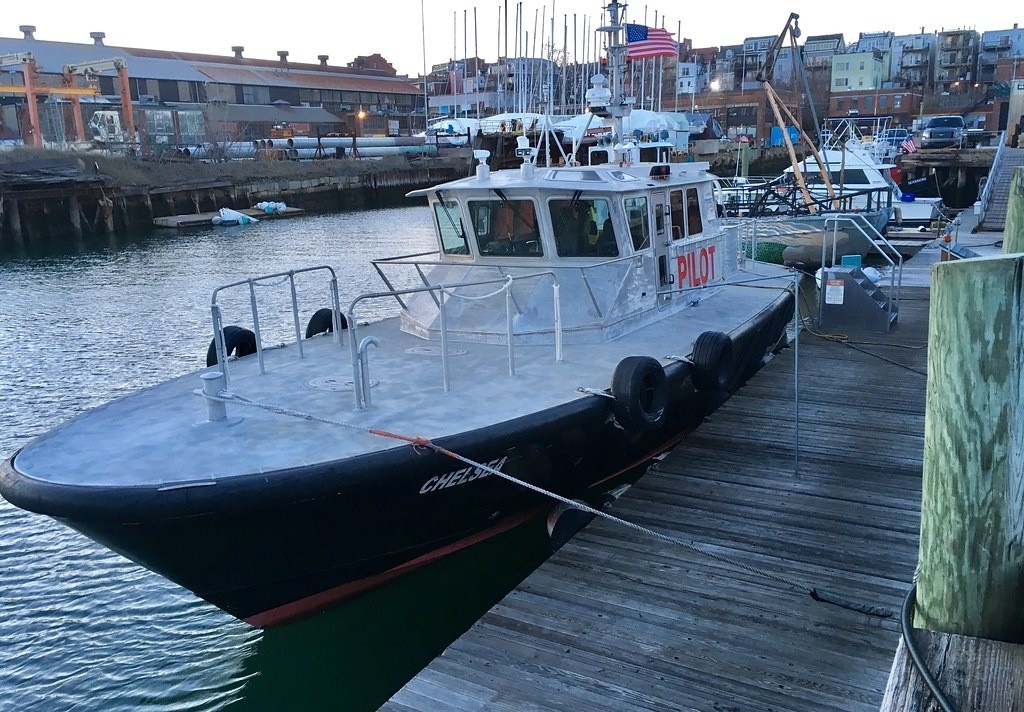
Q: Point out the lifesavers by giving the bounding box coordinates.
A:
[207,325,257,368]
[611,357,667,430]
[306,308,347,339]
[691,330,736,390]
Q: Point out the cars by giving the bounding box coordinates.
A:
[818,130,834,144]
[921,116,968,148]
[873,128,914,154]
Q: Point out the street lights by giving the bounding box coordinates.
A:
[358,105,364,136]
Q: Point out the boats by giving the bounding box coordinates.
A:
[720,149,942,223]
[706,173,896,270]
[581,144,749,207]
[1,140,807,630]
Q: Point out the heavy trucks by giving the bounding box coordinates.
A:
[88,110,206,145]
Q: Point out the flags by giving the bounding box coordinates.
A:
[741,135,748,141]
[627,23,677,61]
[902,136,915,152]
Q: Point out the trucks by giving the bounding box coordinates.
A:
[270,122,311,139]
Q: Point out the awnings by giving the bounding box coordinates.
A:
[207,106,344,123]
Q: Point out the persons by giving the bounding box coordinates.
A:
[499,118,522,133]
